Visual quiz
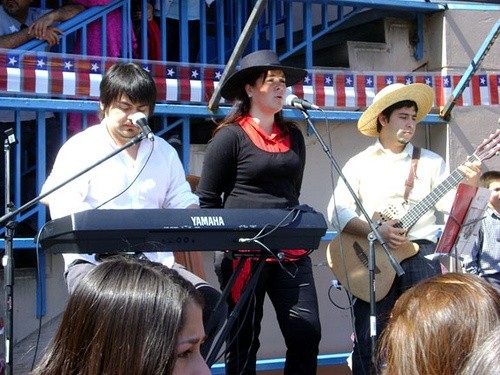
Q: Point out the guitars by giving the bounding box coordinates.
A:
[327,128,500,303]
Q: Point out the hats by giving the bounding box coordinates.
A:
[357,82,435,139]
[219,49,308,101]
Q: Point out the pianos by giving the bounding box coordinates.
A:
[33,205,327,370]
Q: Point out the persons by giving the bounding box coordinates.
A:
[193,50,322,375]
[376,271,499,375]
[327,81,482,375]
[39,63,227,370]
[26,253,213,375]
[461,171,500,296]
[0,0,65,217]
[68,0,216,147]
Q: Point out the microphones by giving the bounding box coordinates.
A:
[286,94,324,112]
[131,111,155,143]
